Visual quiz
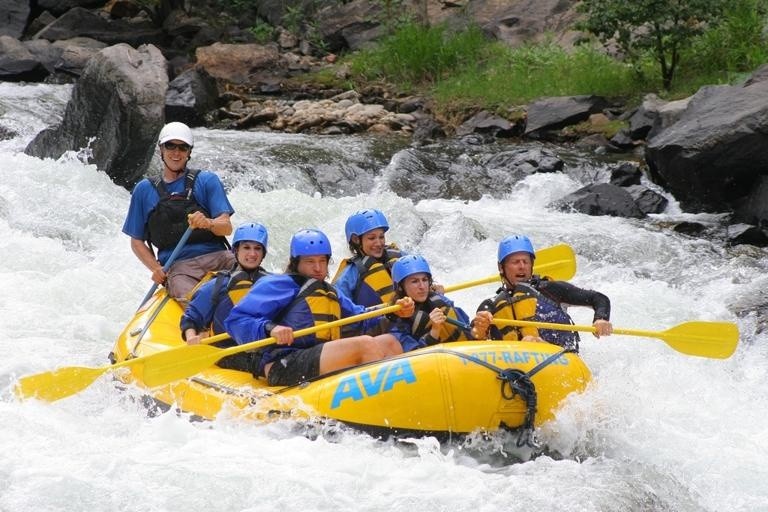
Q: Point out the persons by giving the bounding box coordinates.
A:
[180,223,273,372]
[386,255,473,354]
[331,208,444,337]
[469,233,613,355]
[223,228,404,388]
[121,121,239,313]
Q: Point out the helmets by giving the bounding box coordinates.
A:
[231,222,268,254]
[290,229,331,259]
[344,208,390,244]
[391,254,431,291]
[498,234,536,264]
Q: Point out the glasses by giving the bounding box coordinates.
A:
[164,141,190,151]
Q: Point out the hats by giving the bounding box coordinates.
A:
[159,122,194,147]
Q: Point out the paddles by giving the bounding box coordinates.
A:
[490,318,740,359]
[12,332,231,403]
[444,243,577,294]
[129,304,402,389]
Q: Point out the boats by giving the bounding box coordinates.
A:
[107,265,607,446]
